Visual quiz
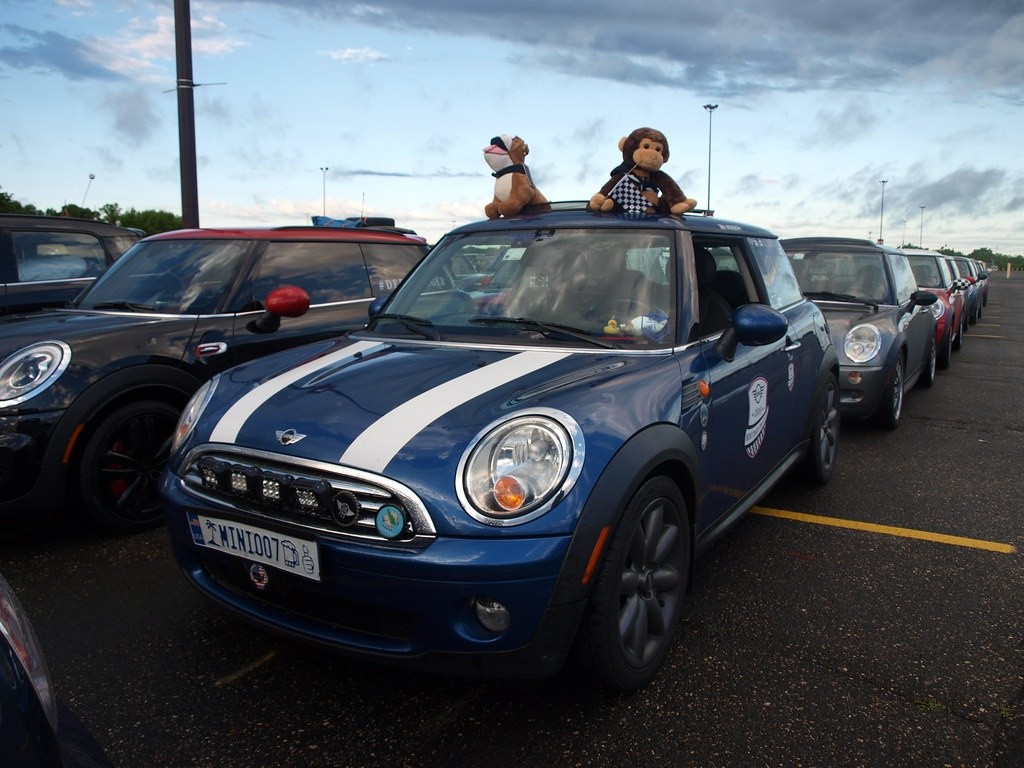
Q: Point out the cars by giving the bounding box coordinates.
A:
[1,205,144,317]
[894,247,991,367]
[1,217,430,519]
[161,205,842,692]
[775,236,938,432]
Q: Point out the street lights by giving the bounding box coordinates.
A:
[919,206,925,249]
[320,167,329,218]
[877,180,888,245]
[703,103,719,209]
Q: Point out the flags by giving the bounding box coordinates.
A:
[608,174,653,215]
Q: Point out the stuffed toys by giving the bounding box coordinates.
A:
[482,134,549,218]
[586,127,697,215]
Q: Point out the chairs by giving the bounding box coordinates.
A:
[517,251,593,331]
[911,265,938,288]
[603,269,649,332]
[855,265,887,303]
[790,259,812,292]
[666,247,737,344]
